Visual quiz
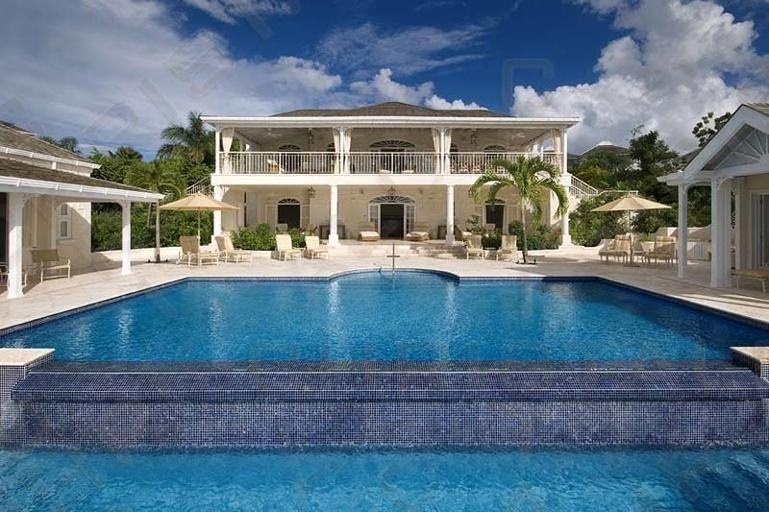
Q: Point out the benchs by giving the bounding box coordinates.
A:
[30,248,72,283]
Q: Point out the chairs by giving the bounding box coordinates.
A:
[466,233,519,262]
[178,234,328,265]
[599,234,676,266]
